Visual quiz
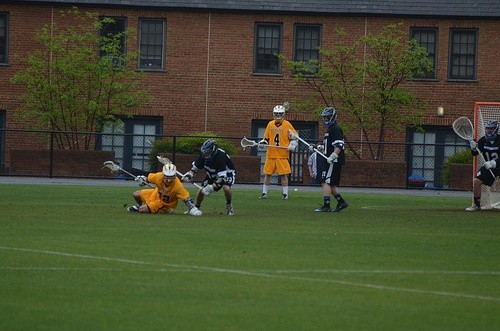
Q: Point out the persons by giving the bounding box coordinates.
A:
[465,119,500,211]
[258,105,299,200]
[127,163,203,216]
[309,107,348,212]
[181,139,237,216]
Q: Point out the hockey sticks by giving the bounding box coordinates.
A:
[451,117,499,185]
[240,138,289,149]
[104,161,155,188]
[289,129,333,164]
[157,156,210,197]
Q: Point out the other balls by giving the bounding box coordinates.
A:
[438,106,444,116]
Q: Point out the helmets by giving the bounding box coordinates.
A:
[321,107,337,127]
[484,120,499,139]
[273,105,287,123]
[200,140,218,161]
[163,163,176,177]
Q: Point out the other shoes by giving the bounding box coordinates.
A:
[314,203,331,212]
[332,200,348,212]
[128,204,142,212]
[225,203,234,216]
[281,194,289,200]
[465,203,482,211]
[258,193,268,199]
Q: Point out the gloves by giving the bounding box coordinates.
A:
[327,152,338,164]
[258,139,269,150]
[135,175,149,187]
[309,143,318,153]
[201,184,215,196]
[189,206,202,217]
[287,140,298,152]
[181,171,193,182]
[470,141,478,151]
[482,160,496,169]
